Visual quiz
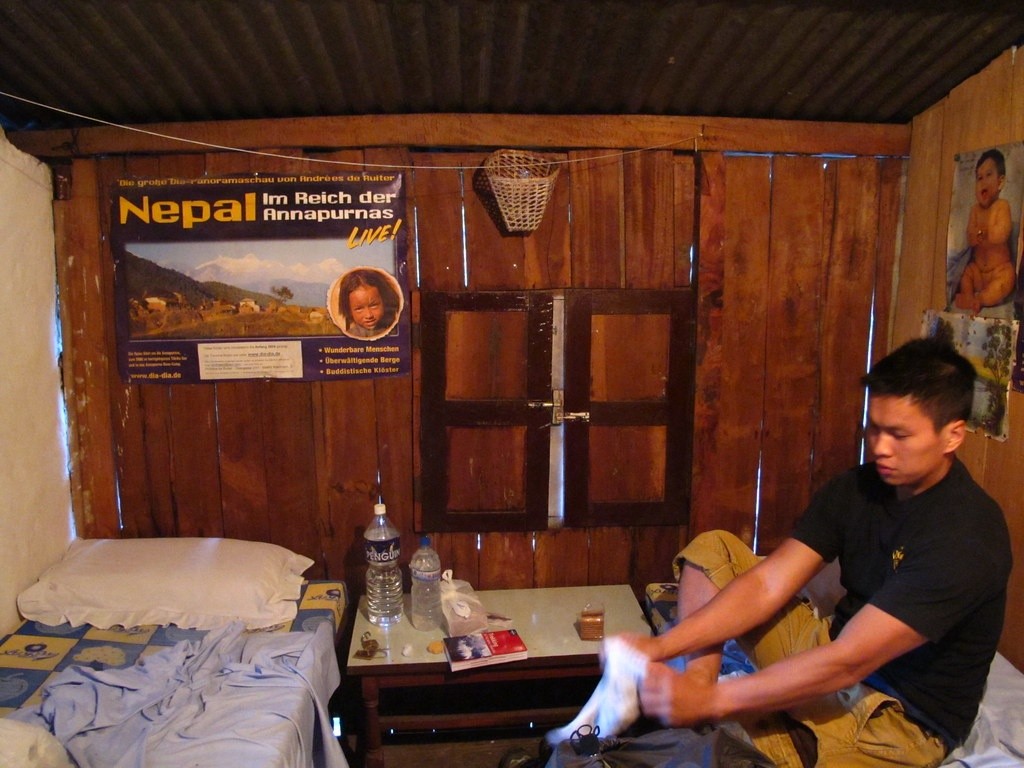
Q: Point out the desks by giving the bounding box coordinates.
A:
[347,583,655,768]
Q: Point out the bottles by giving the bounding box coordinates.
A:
[364,503,404,627]
[409,537,442,631]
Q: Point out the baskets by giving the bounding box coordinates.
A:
[483,149,561,232]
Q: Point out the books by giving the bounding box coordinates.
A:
[442,629,528,671]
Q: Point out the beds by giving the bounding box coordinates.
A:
[644,581,1024,768]
[0,579,351,768]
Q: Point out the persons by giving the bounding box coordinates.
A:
[338,271,400,338]
[601,337,1012,768]
[957,149,1016,312]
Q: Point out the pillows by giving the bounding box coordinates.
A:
[16,535,315,630]
